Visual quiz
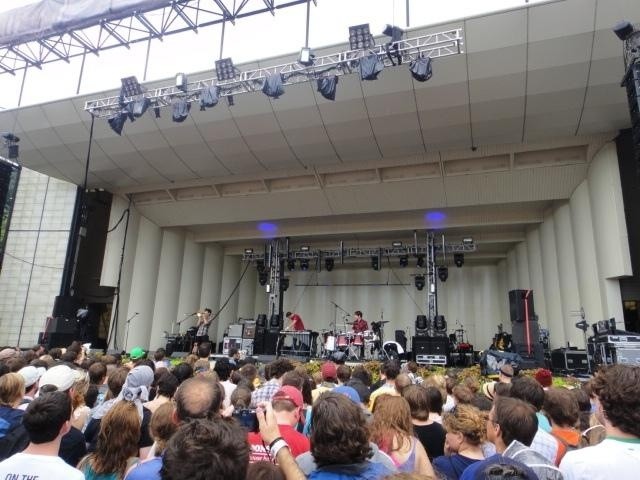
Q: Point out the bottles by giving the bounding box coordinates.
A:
[273,385,305,425]
[130,347,145,359]
[460,440,564,480]
[535,370,552,386]
[323,363,336,381]
[483,381,497,400]
[0,348,20,361]
[18,365,81,393]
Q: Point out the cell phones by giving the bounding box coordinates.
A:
[232,408,266,432]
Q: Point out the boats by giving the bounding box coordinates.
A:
[0,159,21,273]
[38,286,115,349]
[253,332,281,354]
[620,61,640,177]
[614,128,640,279]
[449,352,474,368]
[60,185,112,297]
[508,290,545,370]
[416,315,426,329]
[552,348,588,373]
[271,315,279,326]
[412,335,449,367]
[435,316,444,328]
[256,314,266,326]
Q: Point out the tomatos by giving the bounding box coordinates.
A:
[280,330,312,336]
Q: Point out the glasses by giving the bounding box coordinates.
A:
[245,237,473,291]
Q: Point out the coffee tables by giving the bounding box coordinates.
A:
[347,322,357,325]
[456,329,464,332]
[379,321,389,323]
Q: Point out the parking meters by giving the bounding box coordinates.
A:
[184,313,218,319]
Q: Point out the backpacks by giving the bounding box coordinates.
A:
[337,333,351,348]
[353,333,364,346]
[364,331,373,339]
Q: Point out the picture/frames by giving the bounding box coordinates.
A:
[274,330,313,359]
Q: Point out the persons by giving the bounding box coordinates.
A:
[284,312,304,355]
[0,340,640,480]
[352,310,368,334]
[196,308,211,343]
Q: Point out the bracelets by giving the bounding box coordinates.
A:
[270,440,291,459]
[269,438,284,448]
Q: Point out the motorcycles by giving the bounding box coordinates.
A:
[332,308,391,362]
